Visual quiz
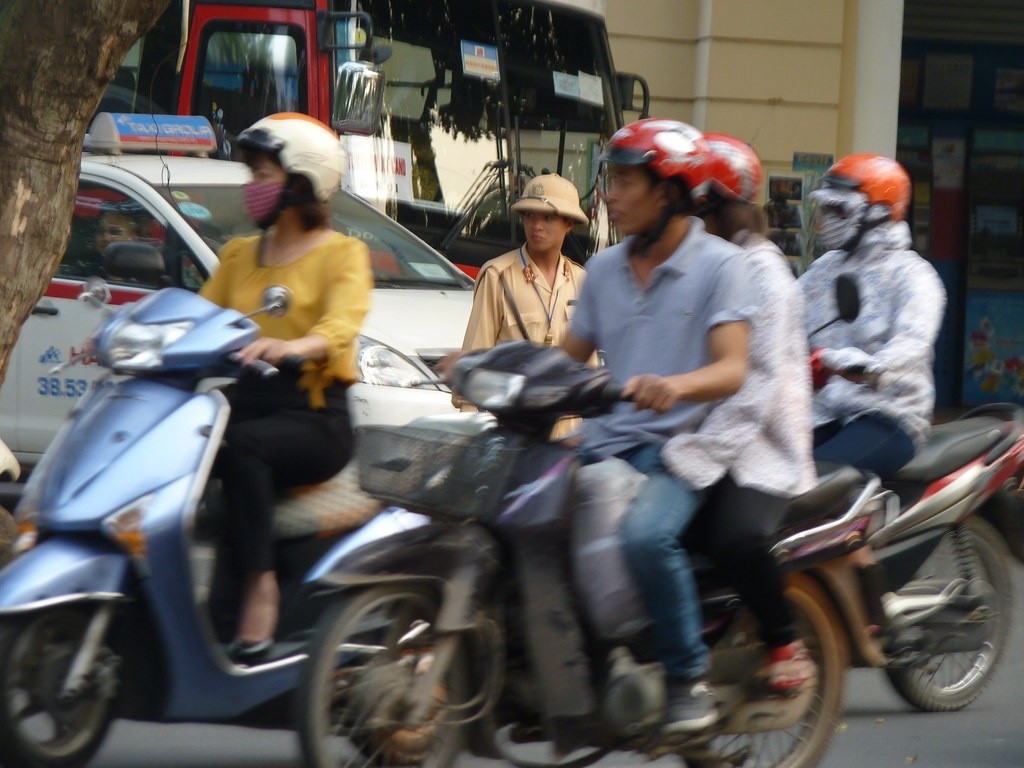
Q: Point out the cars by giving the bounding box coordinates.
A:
[0,112,481,514]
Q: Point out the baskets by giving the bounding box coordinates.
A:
[365,422,519,519]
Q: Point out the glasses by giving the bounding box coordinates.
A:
[818,203,855,219]
[96,226,123,236]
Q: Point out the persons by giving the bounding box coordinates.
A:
[48,208,167,291]
[190,109,374,661]
[450,172,603,442]
[663,130,821,697]
[796,148,946,651]
[435,116,756,736]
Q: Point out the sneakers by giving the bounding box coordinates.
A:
[748,640,820,698]
[666,674,726,734]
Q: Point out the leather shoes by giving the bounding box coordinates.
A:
[229,637,273,662]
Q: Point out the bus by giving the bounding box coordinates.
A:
[91,0,652,282]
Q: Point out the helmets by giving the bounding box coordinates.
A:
[600,119,706,204]
[700,133,767,208]
[819,153,913,221]
[237,110,347,203]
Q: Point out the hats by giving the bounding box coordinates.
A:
[508,172,589,228]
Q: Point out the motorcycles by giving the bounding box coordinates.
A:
[297,339,847,768]
[804,270,1024,711]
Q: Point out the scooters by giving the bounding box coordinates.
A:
[0,271,497,767]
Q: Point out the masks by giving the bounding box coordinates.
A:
[242,179,283,221]
